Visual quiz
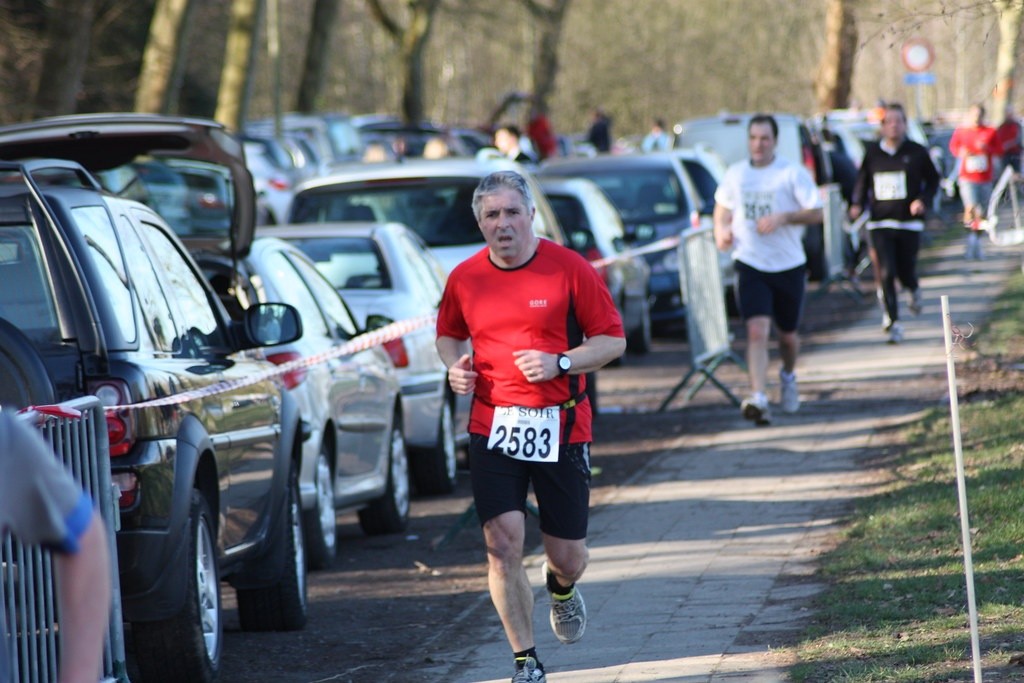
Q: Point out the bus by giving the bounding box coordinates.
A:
[0,156,306,683]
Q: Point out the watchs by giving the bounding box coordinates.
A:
[556,352,572,378]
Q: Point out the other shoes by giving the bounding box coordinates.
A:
[906,287,921,315]
[886,326,902,344]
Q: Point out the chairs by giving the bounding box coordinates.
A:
[637,183,667,207]
[341,202,377,221]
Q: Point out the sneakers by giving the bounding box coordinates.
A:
[541,561,587,644]
[741,399,770,425]
[780,369,799,414]
[511,655,546,683]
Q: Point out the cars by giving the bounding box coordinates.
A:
[0,100,965,572]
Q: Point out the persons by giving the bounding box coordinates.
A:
[713,115,825,428]
[949,105,1004,260]
[494,123,531,164]
[849,104,940,344]
[527,108,560,158]
[0,405,109,683]
[642,118,669,150]
[588,107,611,153]
[997,112,1023,202]
[435,170,627,683]
